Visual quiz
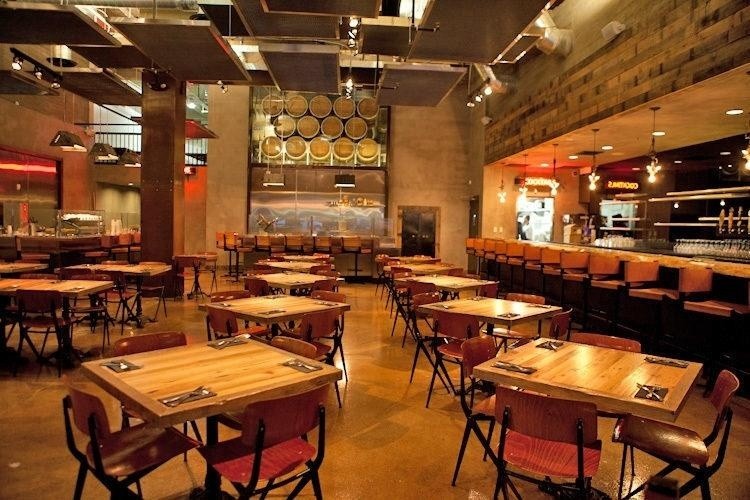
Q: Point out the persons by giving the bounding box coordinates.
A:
[517,209,552,242]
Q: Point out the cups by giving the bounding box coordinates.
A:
[110,219,121,237]
[593,236,636,249]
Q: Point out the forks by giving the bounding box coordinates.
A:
[168,387,211,409]
[646,383,661,399]
[118,362,128,370]
[496,361,531,372]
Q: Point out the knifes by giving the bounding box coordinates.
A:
[218,336,237,350]
[289,360,317,372]
[637,383,663,404]
[170,383,204,407]
[100,363,134,367]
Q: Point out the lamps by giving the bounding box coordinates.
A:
[10,48,64,90]
[88,107,119,161]
[262,87,285,188]
[499,68,750,203]
[334,86,355,188]
[465,78,493,109]
[119,121,143,166]
[50,91,88,153]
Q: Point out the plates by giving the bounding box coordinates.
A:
[261,93,379,161]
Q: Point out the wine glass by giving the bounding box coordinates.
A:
[673,238,750,259]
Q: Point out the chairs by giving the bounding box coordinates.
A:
[0,231,750,500]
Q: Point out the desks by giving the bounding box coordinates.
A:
[254,258,335,272]
[80,245,353,494]
[373,251,712,496]
[2,249,220,379]
